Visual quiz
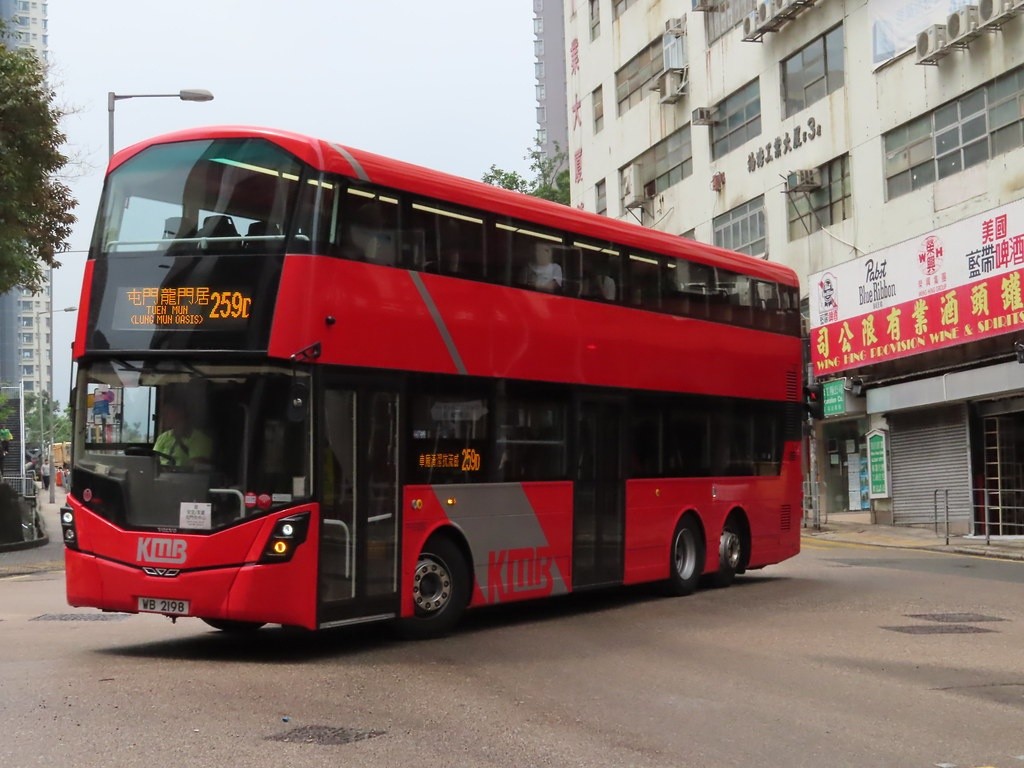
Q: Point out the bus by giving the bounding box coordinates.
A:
[59,124,807,636]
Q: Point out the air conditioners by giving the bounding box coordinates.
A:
[757,1,777,30]
[656,70,688,106]
[786,170,822,192]
[946,4,978,45]
[691,0,714,12]
[915,23,945,62]
[664,17,684,38]
[978,0,1013,30]
[624,187,647,208]
[743,9,759,39]
[772,0,797,17]
[691,107,721,127]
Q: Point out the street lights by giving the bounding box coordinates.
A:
[108,87,215,160]
[35,306,77,486]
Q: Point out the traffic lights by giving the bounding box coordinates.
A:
[803,382,825,420]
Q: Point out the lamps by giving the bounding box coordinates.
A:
[849,376,864,398]
[1013,340,1024,364]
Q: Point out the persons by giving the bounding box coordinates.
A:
[152,390,213,466]
[41,460,49,489]
[581,264,616,299]
[0,424,10,456]
[520,243,562,288]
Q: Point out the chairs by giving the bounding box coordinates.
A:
[155,214,312,252]
[562,265,788,334]
